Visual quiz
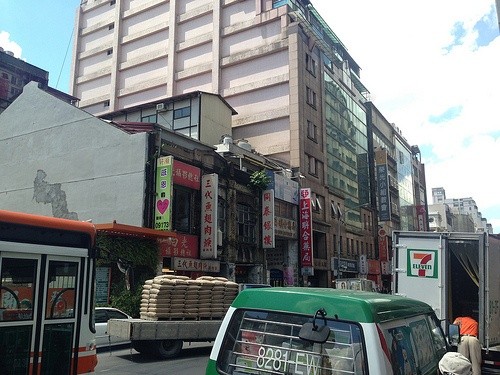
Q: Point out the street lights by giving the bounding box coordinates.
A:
[337,203,370,290]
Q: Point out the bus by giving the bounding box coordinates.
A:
[0,209,100,375]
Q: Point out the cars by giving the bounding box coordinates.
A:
[95,306,137,349]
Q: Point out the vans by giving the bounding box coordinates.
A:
[199,287,453,375]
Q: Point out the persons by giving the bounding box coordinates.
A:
[452,308,482,375]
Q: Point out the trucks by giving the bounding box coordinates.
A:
[390,228,498,369]
[106,274,275,362]
